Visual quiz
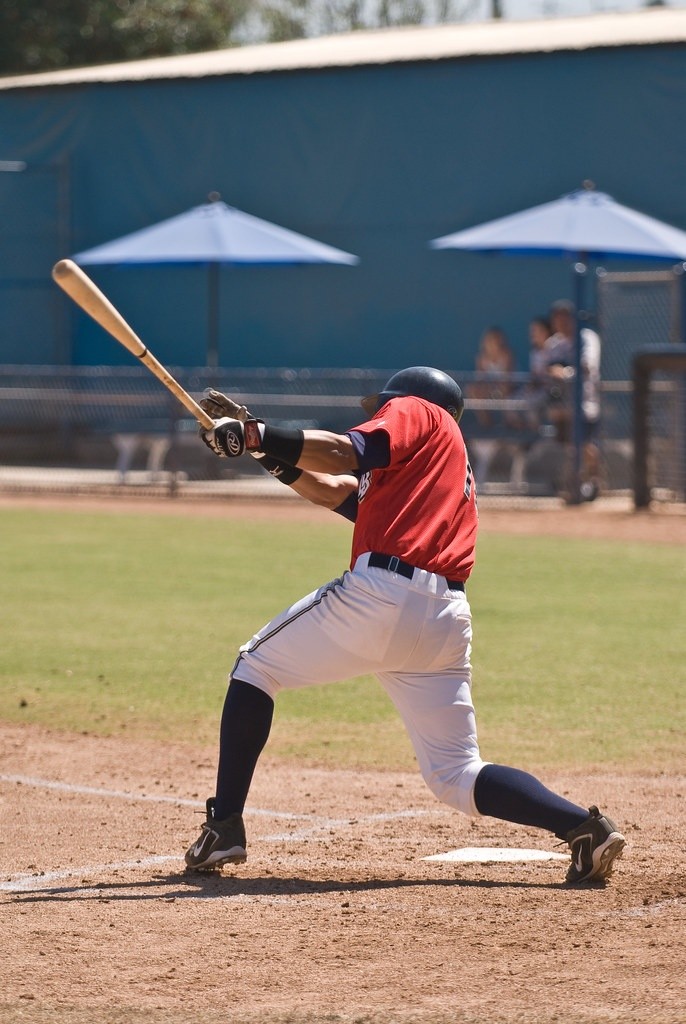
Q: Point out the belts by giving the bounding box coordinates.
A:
[368,553,465,593]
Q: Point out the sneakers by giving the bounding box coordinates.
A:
[552,805,626,885]
[184,798,248,868]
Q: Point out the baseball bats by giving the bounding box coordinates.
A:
[50,259,215,431]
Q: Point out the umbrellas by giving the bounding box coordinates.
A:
[430,188,686,477]
[66,189,359,393]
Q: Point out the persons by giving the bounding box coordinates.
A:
[472,300,603,503]
[185,365,628,882]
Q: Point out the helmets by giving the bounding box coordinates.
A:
[361,367,465,424]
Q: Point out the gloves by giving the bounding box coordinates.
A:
[198,392,303,486]
[200,417,304,468]
